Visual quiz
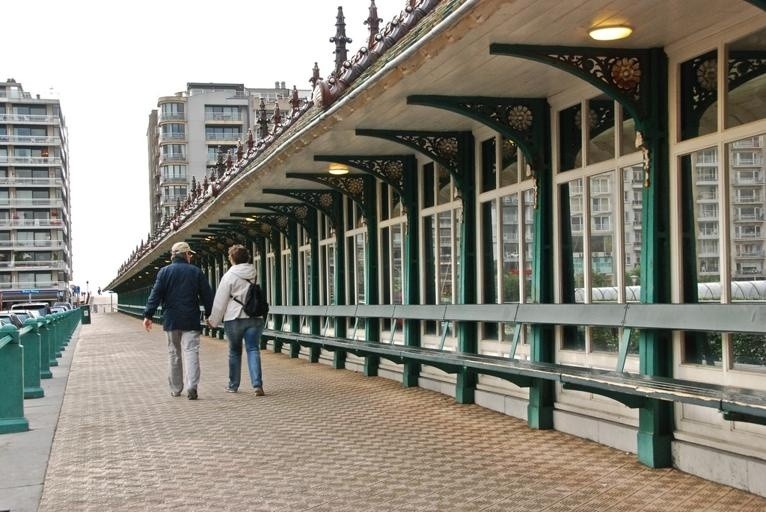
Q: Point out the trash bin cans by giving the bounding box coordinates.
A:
[80,305,91,324]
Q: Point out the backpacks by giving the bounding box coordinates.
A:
[230,275,269,317]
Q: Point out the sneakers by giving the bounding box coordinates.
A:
[253,386,264,397]
[224,386,238,394]
[186,389,198,400]
[169,390,180,398]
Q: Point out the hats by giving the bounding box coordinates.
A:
[171,242,196,255]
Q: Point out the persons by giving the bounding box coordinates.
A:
[206,244,266,395]
[143,243,216,400]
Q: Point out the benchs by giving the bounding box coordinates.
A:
[260,304,766,424]
